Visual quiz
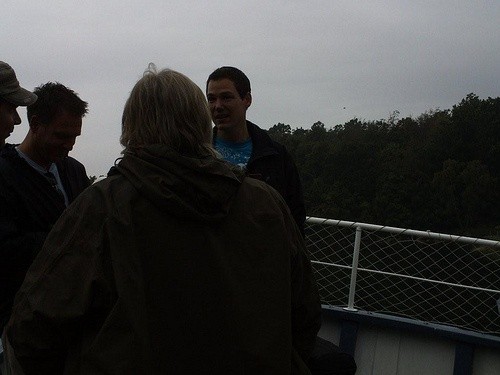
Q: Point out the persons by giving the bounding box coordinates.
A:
[0,70,323,375]
[207,67,305,243]
[0,61,67,340]
[6,82,93,209]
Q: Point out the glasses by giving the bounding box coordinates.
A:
[45,171,66,204]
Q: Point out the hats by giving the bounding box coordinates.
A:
[0,61,38,105]
